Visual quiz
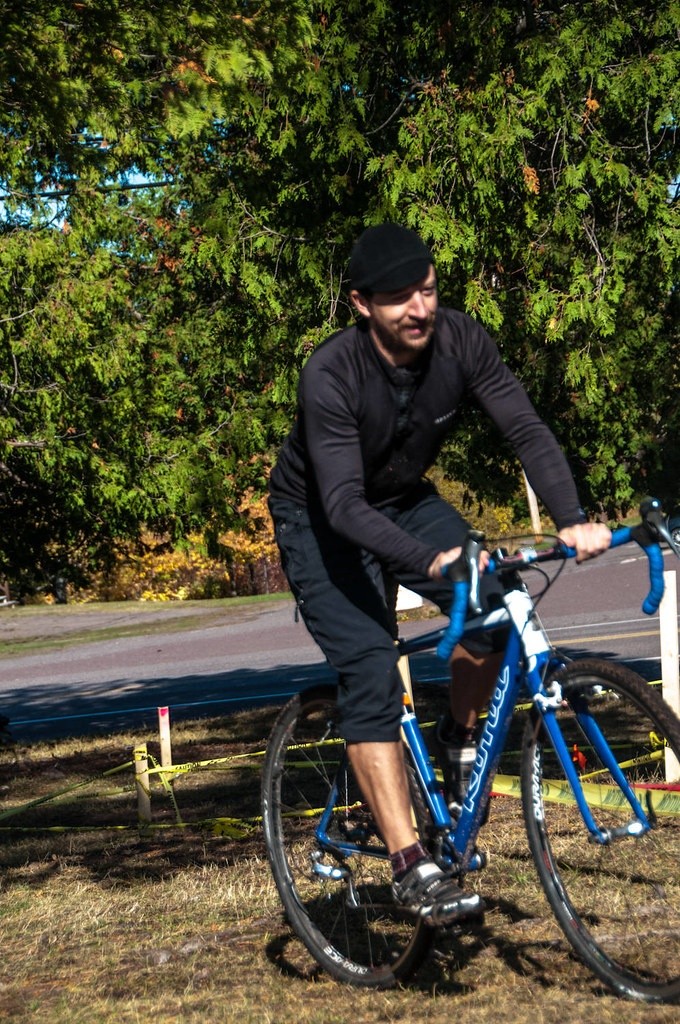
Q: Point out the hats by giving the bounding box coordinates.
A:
[341,224,434,291]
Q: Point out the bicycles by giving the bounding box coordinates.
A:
[260,496,680,1006]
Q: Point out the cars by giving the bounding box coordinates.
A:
[655,503,680,549]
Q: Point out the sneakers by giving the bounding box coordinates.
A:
[431,714,479,825]
[391,860,484,926]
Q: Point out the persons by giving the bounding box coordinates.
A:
[267,223,614,927]
